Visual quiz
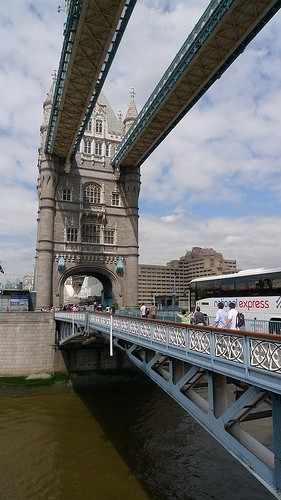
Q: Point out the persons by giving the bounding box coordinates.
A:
[106,305,110,312]
[41,304,80,311]
[109,303,115,314]
[176,309,193,324]
[93,302,98,312]
[224,302,241,330]
[208,302,228,329]
[140,302,149,318]
[98,304,103,312]
[150,303,158,319]
[194,306,206,325]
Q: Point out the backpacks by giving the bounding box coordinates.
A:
[235,311,244,328]
[145,307,150,316]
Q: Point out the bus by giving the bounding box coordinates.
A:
[183,268,281,336]
